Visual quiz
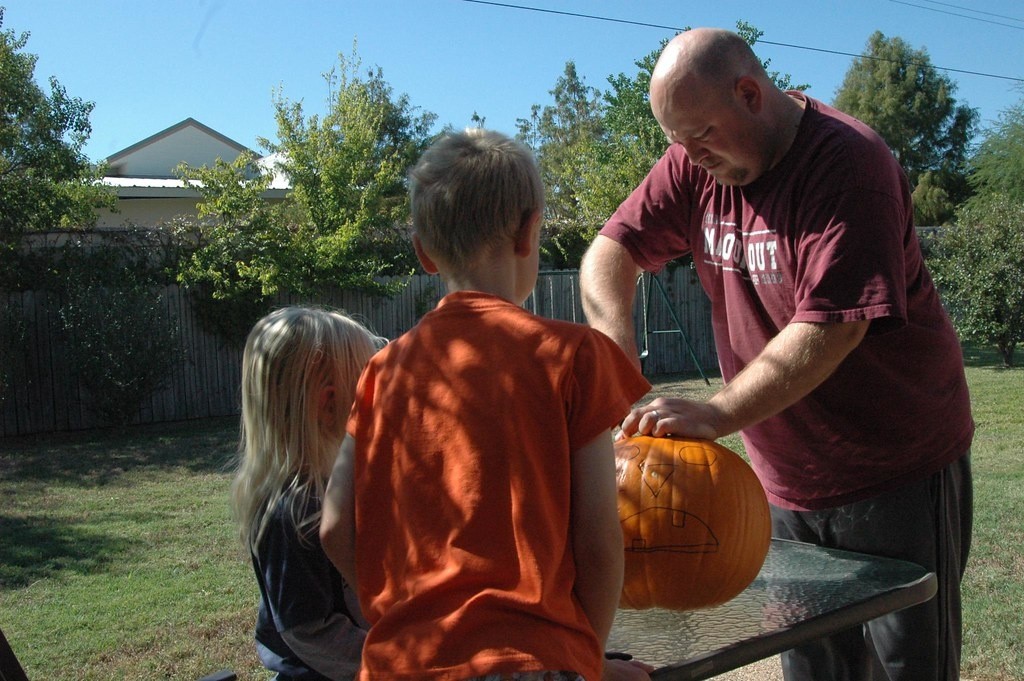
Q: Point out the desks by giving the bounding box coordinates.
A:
[605,537,937,681]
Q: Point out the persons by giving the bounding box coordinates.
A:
[319,127,654,681]
[580,31,976,681]
[230,309,389,681]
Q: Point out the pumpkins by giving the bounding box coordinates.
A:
[612,436,771,611]
